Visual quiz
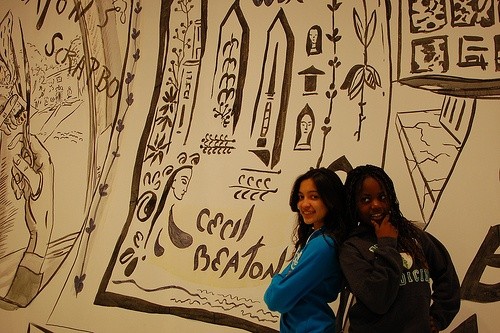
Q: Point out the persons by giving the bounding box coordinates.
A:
[338,165,462,333]
[263,166,348,333]
